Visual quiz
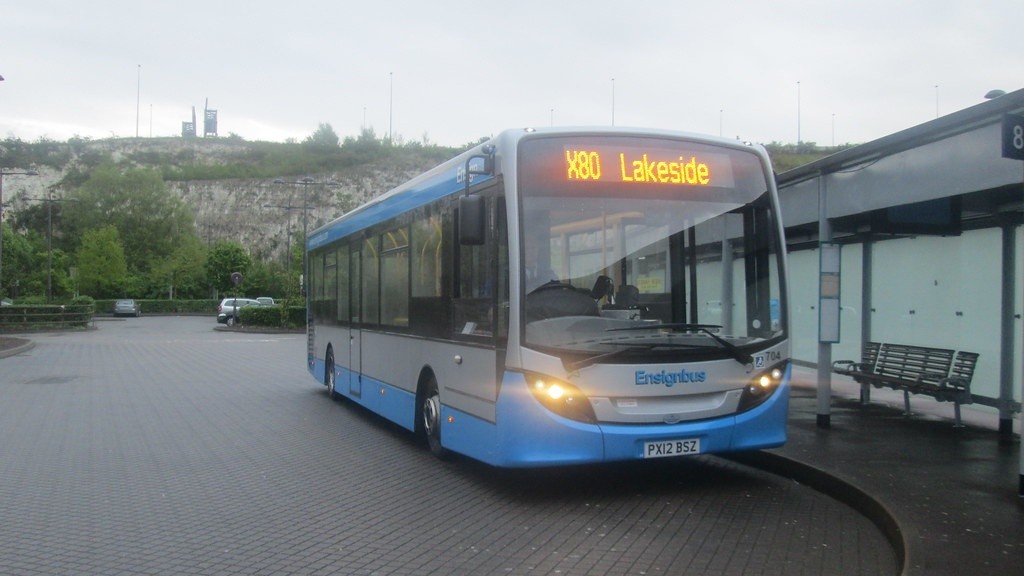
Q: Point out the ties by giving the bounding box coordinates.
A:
[526,266,536,282]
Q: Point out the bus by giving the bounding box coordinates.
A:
[303,125,793,477]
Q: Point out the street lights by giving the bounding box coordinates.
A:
[797,81,801,144]
[611,79,615,129]
[135,65,141,138]
[832,113,835,146]
[388,72,393,143]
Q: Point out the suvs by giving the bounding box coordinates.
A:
[216,297,263,326]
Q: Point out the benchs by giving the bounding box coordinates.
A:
[831,342,979,428]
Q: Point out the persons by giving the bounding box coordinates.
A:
[524,231,558,294]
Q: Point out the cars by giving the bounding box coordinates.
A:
[256,297,275,307]
[112,298,141,317]
